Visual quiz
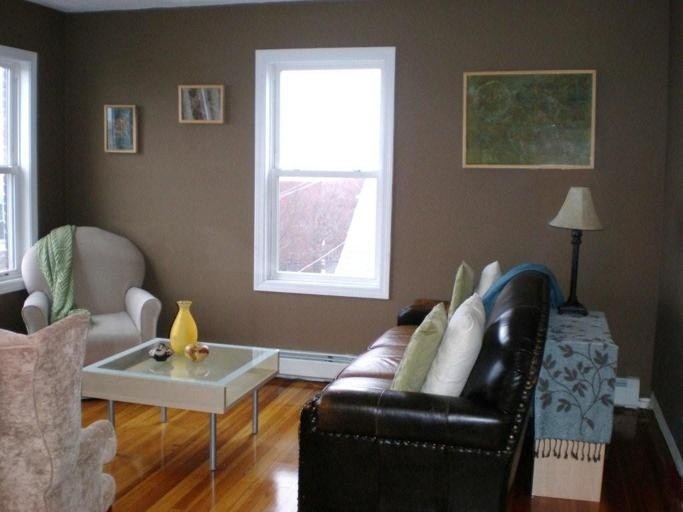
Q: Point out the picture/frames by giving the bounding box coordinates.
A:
[103,105,138,154]
[462,69,597,170]
[177,84,226,125]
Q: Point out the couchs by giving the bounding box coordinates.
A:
[0,310,116,512]
[20,227,162,400]
[297,259,550,511]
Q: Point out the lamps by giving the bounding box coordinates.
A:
[548,186,604,316]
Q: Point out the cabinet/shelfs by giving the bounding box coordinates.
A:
[532,308,620,505]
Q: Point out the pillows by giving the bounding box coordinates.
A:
[421,292,486,398]
[389,301,447,392]
[477,260,502,297]
[447,260,475,319]
